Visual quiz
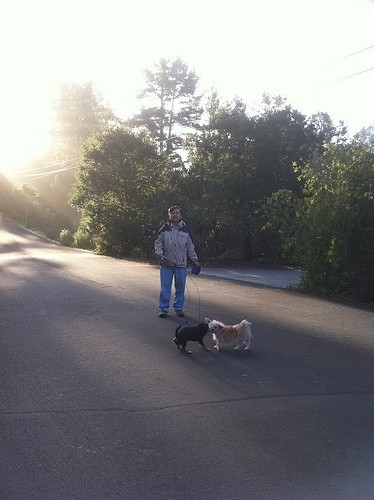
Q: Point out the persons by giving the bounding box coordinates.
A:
[153,205,201,317]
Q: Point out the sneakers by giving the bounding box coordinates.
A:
[175,312,185,317]
[158,312,167,317]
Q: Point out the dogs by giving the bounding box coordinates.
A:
[174,322,210,355]
[205,318,253,351]
